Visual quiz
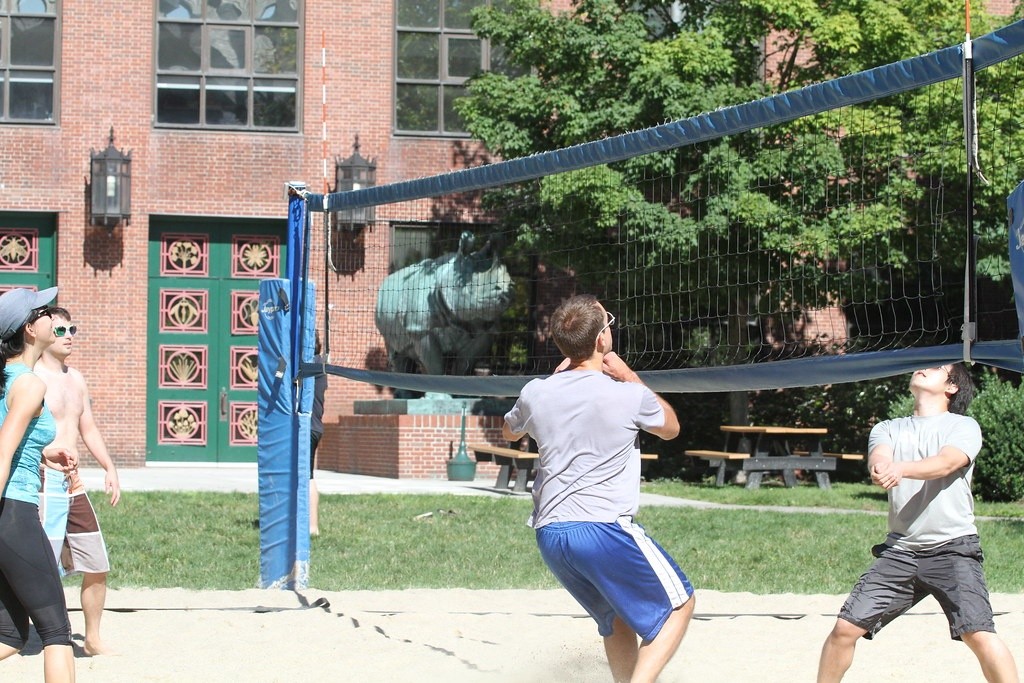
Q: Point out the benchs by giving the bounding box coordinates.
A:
[469,444,539,492]
[796,451,865,489]
[640,454,658,459]
[684,448,750,489]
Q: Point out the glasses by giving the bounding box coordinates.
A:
[53,325,77,337]
[594,312,615,344]
[932,364,957,385]
[20,306,52,333]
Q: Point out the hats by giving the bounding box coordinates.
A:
[0,286,58,341]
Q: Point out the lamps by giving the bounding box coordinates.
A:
[89,127,132,226]
[335,134,379,233]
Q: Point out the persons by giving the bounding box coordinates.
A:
[816,361,1019,683]
[31,307,122,656]
[502,294,696,683]
[309,330,327,535]
[0,286,75,683]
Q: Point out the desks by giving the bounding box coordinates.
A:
[721,425,828,489]
